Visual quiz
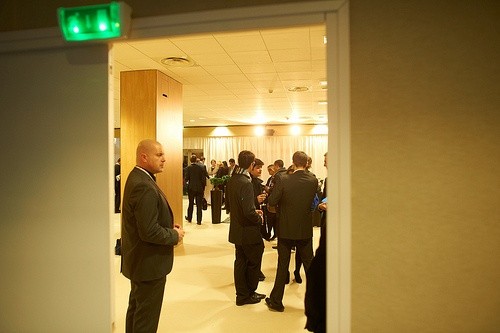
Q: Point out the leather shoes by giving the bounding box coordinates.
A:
[237,292,266,306]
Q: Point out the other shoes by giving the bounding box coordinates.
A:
[265,297,284,312]
[198,223,201,225]
[286,271,290,283]
[258,271,265,281]
[294,271,303,283]
[185,216,191,222]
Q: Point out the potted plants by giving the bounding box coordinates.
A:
[222,175,231,186]
[210,178,223,190]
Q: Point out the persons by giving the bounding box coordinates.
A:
[267,151,319,311]
[226,150,266,306]
[265,156,322,284]
[114,158,121,213]
[196,157,210,209]
[249,158,266,281]
[185,155,206,225]
[120,139,186,333]
[208,159,239,214]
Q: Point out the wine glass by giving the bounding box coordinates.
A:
[261,189,267,207]
